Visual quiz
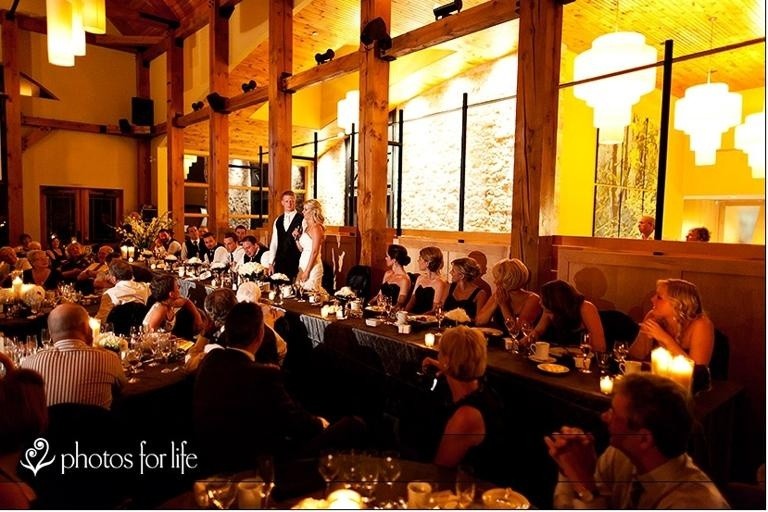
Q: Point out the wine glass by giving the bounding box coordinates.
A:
[208,481,236,510]
[358,459,378,508]
[578,329,592,374]
[119,325,157,352]
[382,454,403,508]
[435,303,444,328]
[160,337,172,375]
[126,349,141,384]
[522,319,534,358]
[508,323,520,355]
[384,296,392,325]
[344,454,362,491]
[613,340,628,368]
[168,337,179,371]
[319,453,340,498]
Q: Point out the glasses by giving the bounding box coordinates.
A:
[35,256,49,260]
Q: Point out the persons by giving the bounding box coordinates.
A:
[0,245,18,341]
[542,372,734,509]
[686,227,709,243]
[143,275,203,342]
[93,259,150,332]
[202,232,231,271]
[186,288,288,378]
[444,258,487,328]
[13,234,42,258]
[420,324,537,504]
[0,368,48,510]
[192,301,325,476]
[367,245,412,309]
[15,250,64,348]
[404,247,449,316]
[120,224,141,259]
[154,229,182,264]
[512,280,610,354]
[476,257,542,340]
[182,225,208,263]
[292,199,325,294]
[268,190,304,284]
[242,236,270,278]
[627,279,721,379]
[223,231,245,274]
[234,225,246,246]
[20,303,127,415]
[45,234,122,284]
[635,215,656,242]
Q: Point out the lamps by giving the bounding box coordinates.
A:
[338,90,359,135]
[573,2,657,144]
[673,16,743,165]
[46,0,106,68]
[735,113,766,179]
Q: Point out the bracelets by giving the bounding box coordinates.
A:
[305,269,310,273]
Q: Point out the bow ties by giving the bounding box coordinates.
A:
[209,249,214,257]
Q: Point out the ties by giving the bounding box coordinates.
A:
[249,257,253,263]
[231,253,233,262]
[286,214,290,227]
[625,480,645,510]
[194,240,197,246]
[93,263,103,271]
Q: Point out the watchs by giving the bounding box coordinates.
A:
[573,489,601,502]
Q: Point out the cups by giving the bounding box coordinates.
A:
[531,341,549,360]
[350,301,360,310]
[408,481,429,509]
[620,361,642,376]
[396,310,407,323]
[238,479,264,510]
[482,488,530,510]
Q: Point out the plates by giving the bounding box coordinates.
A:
[528,354,558,363]
[537,363,570,375]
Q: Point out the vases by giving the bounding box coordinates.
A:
[142,250,152,272]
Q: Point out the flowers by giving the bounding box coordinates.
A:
[108,203,177,254]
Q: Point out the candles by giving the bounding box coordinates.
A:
[652,348,694,393]
[298,489,362,510]
[601,378,612,393]
